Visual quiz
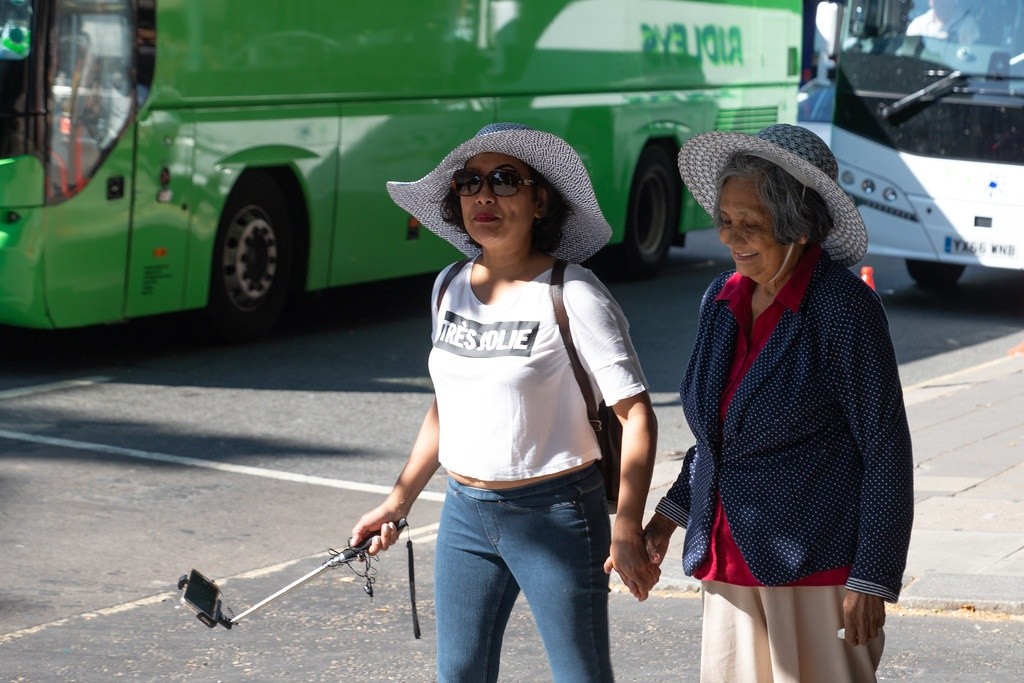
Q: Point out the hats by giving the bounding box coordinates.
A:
[678,123,868,269]
[386,123,613,265]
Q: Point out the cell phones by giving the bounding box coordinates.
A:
[181,569,219,626]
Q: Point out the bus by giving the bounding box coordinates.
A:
[814,1,1024,293]
[0,0,804,351]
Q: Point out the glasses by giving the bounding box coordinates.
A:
[450,167,540,197]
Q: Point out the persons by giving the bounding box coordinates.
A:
[603,122,914,683]
[350,122,663,683]
[800,54,818,87]
[906,0,982,65]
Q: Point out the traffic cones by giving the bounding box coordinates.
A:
[860,266,876,291]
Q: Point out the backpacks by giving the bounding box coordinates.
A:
[436,258,623,506]
